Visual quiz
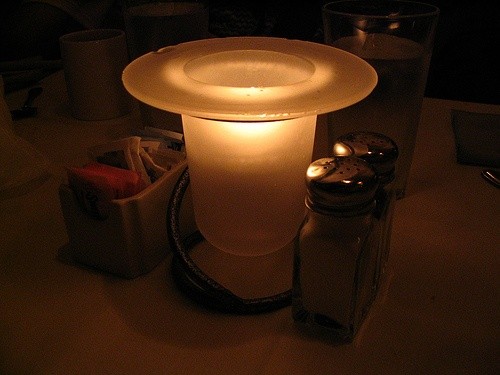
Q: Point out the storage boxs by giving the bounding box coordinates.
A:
[59,150,197,278]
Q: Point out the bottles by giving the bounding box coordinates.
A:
[292,131,399,343]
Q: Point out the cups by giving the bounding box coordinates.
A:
[122,0,209,130]
[59,29,130,121]
[323,0,439,200]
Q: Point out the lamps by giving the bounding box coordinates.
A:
[122,36,378,312]
